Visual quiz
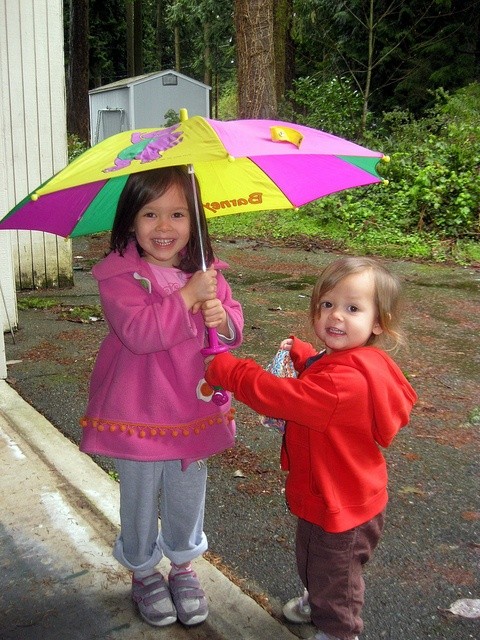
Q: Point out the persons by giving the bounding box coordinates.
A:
[202,255,418,640]
[77,165,246,628]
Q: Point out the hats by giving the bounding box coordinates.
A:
[256,348,299,433]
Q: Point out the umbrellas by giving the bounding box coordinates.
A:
[0,106,386,408]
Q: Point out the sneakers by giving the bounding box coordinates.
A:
[168,565,210,626]
[134,570,179,627]
[304,629,359,640]
[283,589,314,624]
